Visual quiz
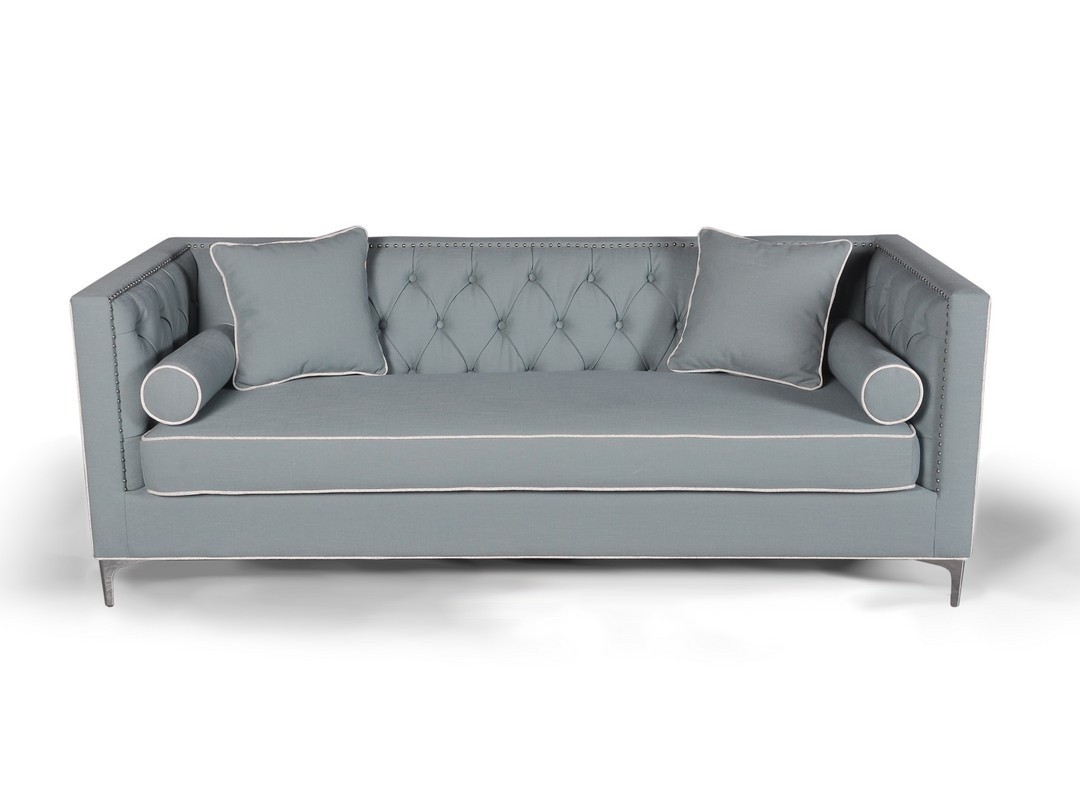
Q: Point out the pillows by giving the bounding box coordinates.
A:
[665,226,854,391]
[139,323,235,426]
[208,226,388,392]
[824,317,924,426]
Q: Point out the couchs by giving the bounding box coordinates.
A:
[69,235,992,609]
